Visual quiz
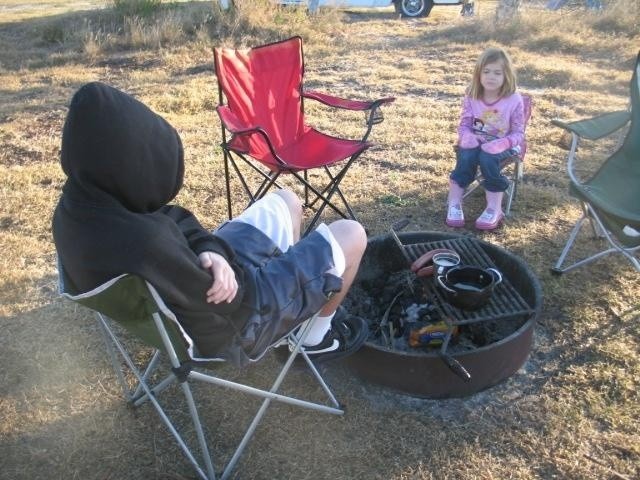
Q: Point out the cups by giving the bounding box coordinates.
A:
[431,253,461,284]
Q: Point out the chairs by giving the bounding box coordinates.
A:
[56,255,347,480]
[549,50,640,279]
[211,37,396,238]
[462,94,533,215]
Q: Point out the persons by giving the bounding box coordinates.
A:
[444,45,528,230]
[49,80,372,369]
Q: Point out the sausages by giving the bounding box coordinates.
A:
[410,248,457,277]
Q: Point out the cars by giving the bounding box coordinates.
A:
[217,0,475,19]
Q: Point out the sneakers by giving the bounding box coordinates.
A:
[274,304,348,360]
[287,316,370,365]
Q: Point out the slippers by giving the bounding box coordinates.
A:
[475,207,505,230]
[446,202,466,228]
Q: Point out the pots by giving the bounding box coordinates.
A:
[438,265,504,311]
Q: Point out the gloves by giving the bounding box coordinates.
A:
[480,137,510,155]
[459,131,486,149]
